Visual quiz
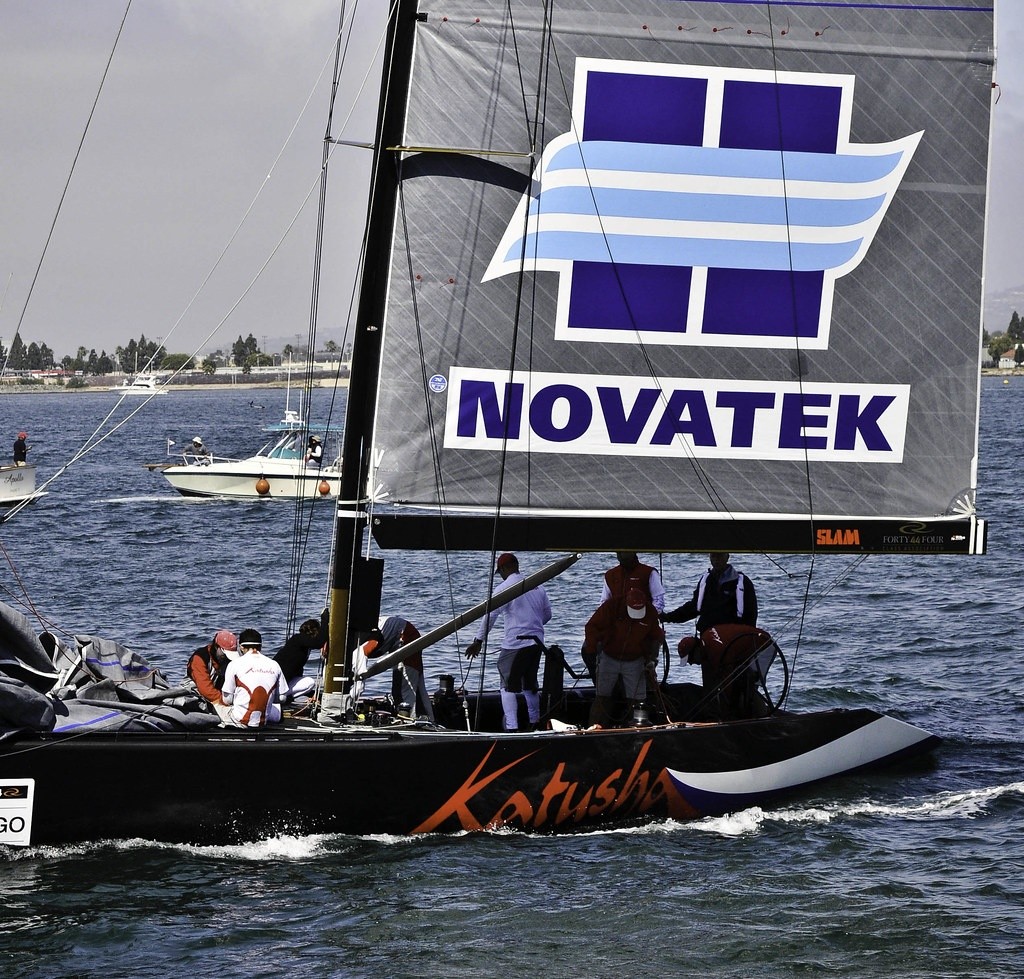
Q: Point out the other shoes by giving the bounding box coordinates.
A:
[754,703,767,718]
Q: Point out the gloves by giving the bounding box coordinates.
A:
[465,637,483,660]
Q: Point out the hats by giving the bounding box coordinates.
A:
[311,435,322,443]
[494,553,519,574]
[215,631,241,661]
[677,637,695,665]
[625,588,647,620]
[192,436,203,444]
[17,432,27,438]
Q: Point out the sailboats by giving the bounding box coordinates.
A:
[2,0,1021,838]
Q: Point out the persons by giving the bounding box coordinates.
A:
[185,631,240,705]
[584,588,665,728]
[351,615,434,721]
[181,436,210,466]
[272,608,329,705]
[600,552,670,723]
[465,554,552,732]
[214,628,290,730]
[306,435,323,467]
[13,431,32,467]
[658,552,758,707]
[678,624,777,720]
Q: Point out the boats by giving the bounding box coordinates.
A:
[165,414,346,504]
[118,372,167,395]
[1,464,49,506]
